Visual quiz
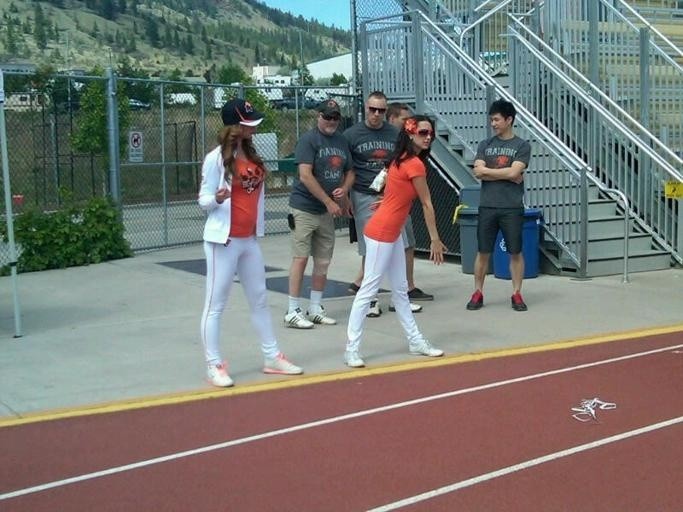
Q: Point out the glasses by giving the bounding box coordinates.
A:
[366,104,389,115]
[320,113,343,123]
[407,128,435,138]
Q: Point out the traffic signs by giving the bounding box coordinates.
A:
[127,130,144,164]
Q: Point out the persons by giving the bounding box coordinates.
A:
[345,114,446,368]
[346,93,423,320]
[284,100,356,331]
[197,99,303,388]
[466,99,530,313]
[347,101,435,306]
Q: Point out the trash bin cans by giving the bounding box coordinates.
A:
[492,208,543,279]
[457,184,493,274]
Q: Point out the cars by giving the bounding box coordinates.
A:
[3,94,50,115]
[271,94,324,111]
[128,99,152,110]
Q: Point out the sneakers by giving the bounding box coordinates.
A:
[511,294,527,312]
[343,351,365,368]
[348,283,360,295]
[205,363,236,388]
[262,355,305,376]
[409,342,444,357]
[408,288,434,302]
[365,297,381,317]
[389,300,422,313]
[466,290,483,311]
[306,310,337,325]
[285,312,313,329]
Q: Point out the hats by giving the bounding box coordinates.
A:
[220,100,266,126]
[316,100,342,117]
